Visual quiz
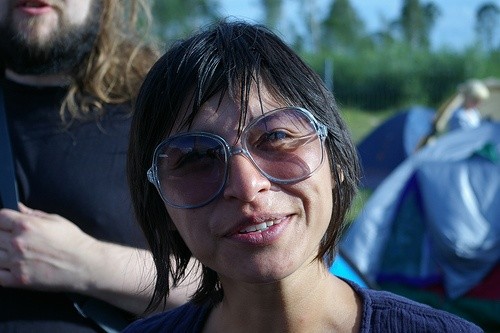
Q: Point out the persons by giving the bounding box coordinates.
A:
[121,22,485,333]
[447,79,489,132]
[0,0,205,333]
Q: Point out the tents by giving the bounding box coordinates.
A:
[337,123,499,300]
[355,105,500,192]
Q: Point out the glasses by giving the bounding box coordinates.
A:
[147,106,328,210]
